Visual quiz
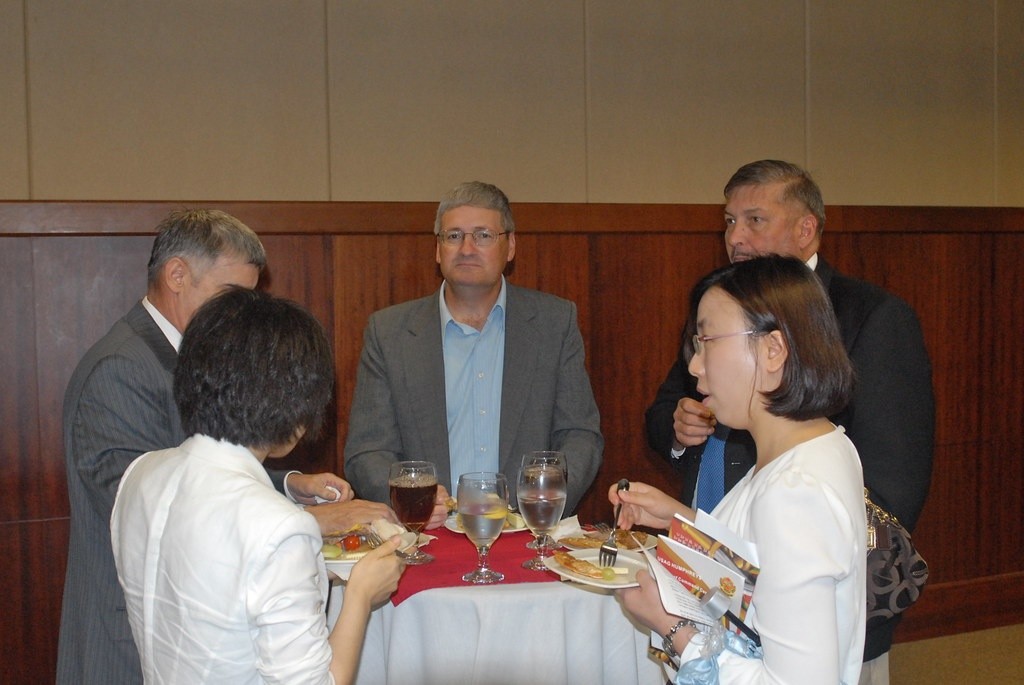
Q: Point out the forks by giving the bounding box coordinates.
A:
[367,532,408,558]
[580,520,610,532]
[598,479,631,566]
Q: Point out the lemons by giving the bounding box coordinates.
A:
[483,506,508,519]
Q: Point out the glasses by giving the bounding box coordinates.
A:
[693,331,758,356]
[436,229,508,246]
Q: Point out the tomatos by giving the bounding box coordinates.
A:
[344,535,360,550]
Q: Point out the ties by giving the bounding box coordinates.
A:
[697,423,732,514]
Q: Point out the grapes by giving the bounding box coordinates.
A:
[601,567,616,580]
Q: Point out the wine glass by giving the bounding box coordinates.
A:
[457,471,509,584]
[521,450,569,550]
[388,460,438,565]
[516,465,567,572]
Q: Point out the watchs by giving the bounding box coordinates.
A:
[663,619,697,656]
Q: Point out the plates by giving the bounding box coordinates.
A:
[544,548,650,588]
[562,529,659,552]
[325,531,417,564]
[444,513,529,534]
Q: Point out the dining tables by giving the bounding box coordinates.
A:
[327,522,670,685]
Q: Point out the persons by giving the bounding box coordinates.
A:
[109,285,406,685]
[342,180,603,530]
[609,253,868,685]
[56,210,406,685]
[644,159,934,685]
